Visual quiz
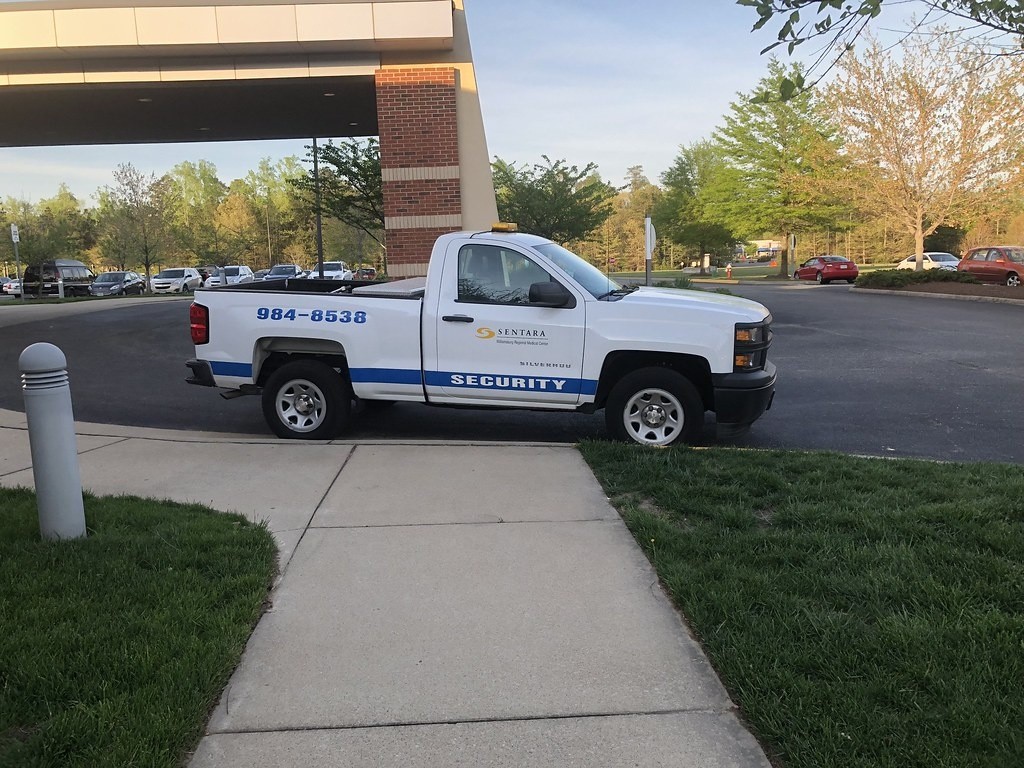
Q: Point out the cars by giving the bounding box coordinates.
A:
[352,268,376,280]
[793,255,859,284]
[0,277,24,298]
[308,261,354,281]
[262,264,307,281]
[896,252,960,272]
[204,266,255,288]
[150,268,204,294]
[87,271,146,296]
[957,245,1024,287]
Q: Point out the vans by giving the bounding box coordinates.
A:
[23,259,99,298]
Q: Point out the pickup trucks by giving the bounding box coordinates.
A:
[183,222,778,449]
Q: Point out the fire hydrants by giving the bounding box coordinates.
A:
[725,264,733,279]
